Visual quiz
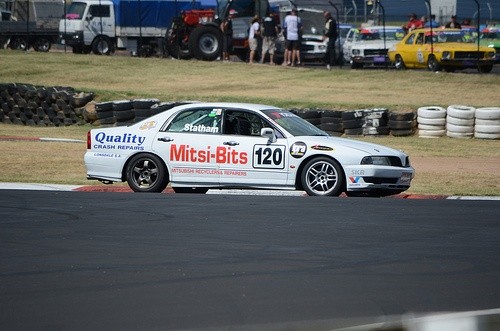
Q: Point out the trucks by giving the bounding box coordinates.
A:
[0,0,200,55]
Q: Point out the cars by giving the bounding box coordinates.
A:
[165,0,405,69]
[84,102,415,196]
[388,28,496,73]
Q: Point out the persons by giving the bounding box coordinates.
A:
[247,7,305,66]
[323,11,338,66]
[220,13,233,63]
[402,14,461,35]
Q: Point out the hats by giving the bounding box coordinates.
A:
[229,9,238,15]
[408,13,417,18]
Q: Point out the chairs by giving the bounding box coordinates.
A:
[235,116,252,136]
